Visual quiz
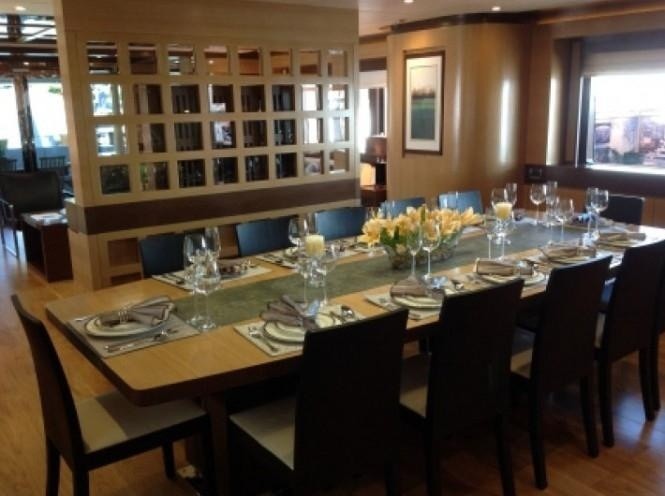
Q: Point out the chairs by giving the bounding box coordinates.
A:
[0,156,74,258]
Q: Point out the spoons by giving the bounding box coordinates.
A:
[330,305,358,324]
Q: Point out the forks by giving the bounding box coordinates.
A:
[248,326,279,353]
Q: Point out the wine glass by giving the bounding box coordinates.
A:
[362,199,396,255]
[183,225,222,331]
[288,218,309,265]
[484,182,518,264]
[405,222,441,284]
[529,180,574,247]
[584,187,608,241]
[295,239,338,318]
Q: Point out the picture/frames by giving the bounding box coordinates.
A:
[402,49,445,159]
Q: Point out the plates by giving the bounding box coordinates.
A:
[83,310,167,337]
[263,311,336,345]
[481,270,545,286]
[390,285,459,310]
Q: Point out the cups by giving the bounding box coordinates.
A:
[446,190,463,211]
